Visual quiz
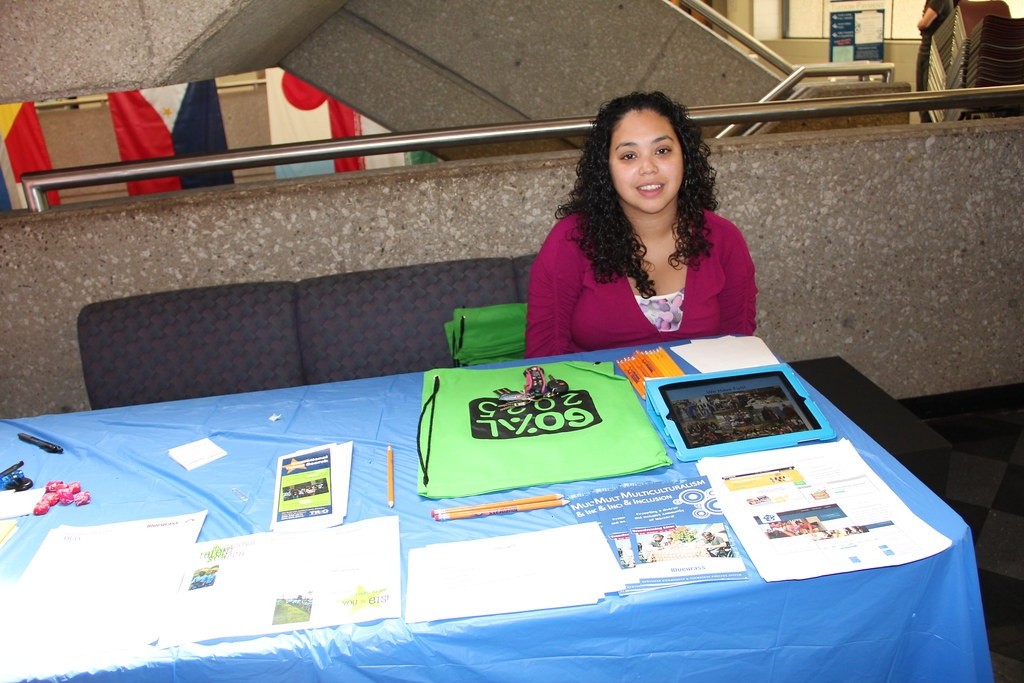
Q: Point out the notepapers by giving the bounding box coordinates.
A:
[167,436,228,471]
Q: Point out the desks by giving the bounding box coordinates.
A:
[0,331,998,682]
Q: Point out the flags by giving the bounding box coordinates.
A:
[265,66,406,180]
[0,101,61,211]
[107,79,235,196]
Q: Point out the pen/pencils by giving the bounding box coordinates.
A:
[616,345,686,402]
[17,430,64,454]
[431,493,571,521]
[0,460,24,479]
[387,445,397,509]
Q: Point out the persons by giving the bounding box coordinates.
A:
[206,568,219,586]
[917,0,961,125]
[768,523,775,534]
[191,569,207,589]
[702,532,726,557]
[525,92,758,360]
[776,517,819,537]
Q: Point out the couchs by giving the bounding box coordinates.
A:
[78,250,540,412]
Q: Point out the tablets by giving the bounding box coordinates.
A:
[645,363,836,462]
